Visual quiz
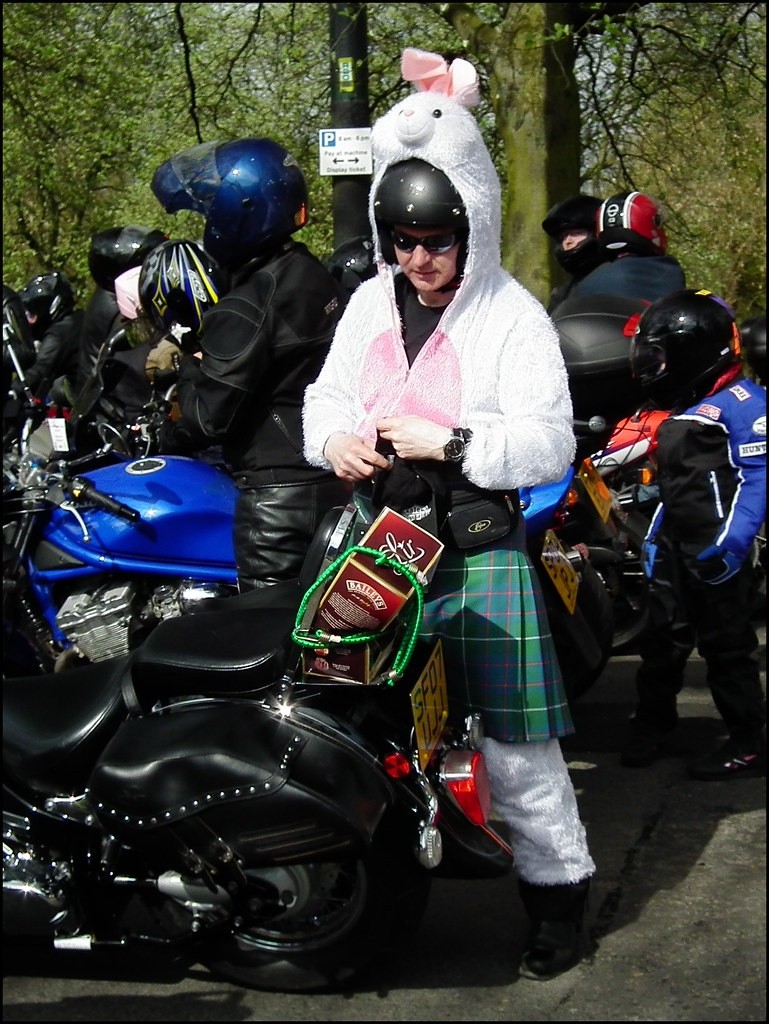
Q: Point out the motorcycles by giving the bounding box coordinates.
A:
[0,284,685,996]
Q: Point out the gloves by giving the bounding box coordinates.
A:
[639,541,659,577]
[697,544,745,585]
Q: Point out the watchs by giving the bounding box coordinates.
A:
[441,426,465,465]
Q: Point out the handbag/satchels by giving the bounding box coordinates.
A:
[434,485,521,550]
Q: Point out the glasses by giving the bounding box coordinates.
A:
[388,230,461,254]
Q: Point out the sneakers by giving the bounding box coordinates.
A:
[619,730,679,767]
[687,742,766,781]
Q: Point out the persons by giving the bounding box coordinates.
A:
[148,138,342,593]
[544,190,669,272]
[3,231,172,450]
[622,287,767,780]
[299,50,594,979]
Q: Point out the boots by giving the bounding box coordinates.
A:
[515,878,590,979]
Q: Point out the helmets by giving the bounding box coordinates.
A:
[151,131,311,274]
[544,195,619,273]
[326,237,379,296]
[630,289,741,404]
[15,274,75,339]
[141,238,228,329]
[377,157,468,273]
[89,227,162,293]
[597,192,669,255]
